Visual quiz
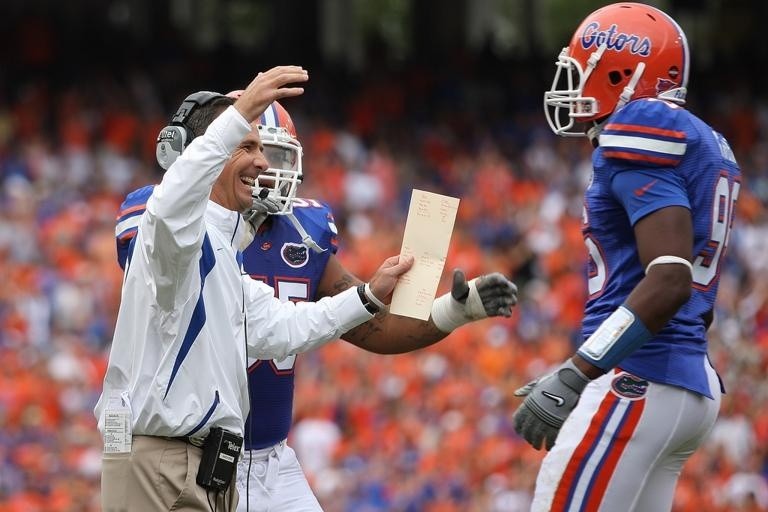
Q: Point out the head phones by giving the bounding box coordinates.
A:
[155,90,227,172]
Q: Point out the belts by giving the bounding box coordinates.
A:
[138,433,205,448]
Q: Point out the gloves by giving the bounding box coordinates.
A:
[509,356,591,452]
[430,267,520,334]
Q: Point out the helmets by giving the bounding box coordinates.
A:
[541,1,691,139]
[223,88,306,218]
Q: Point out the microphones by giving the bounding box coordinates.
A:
[251,188,269,199]
